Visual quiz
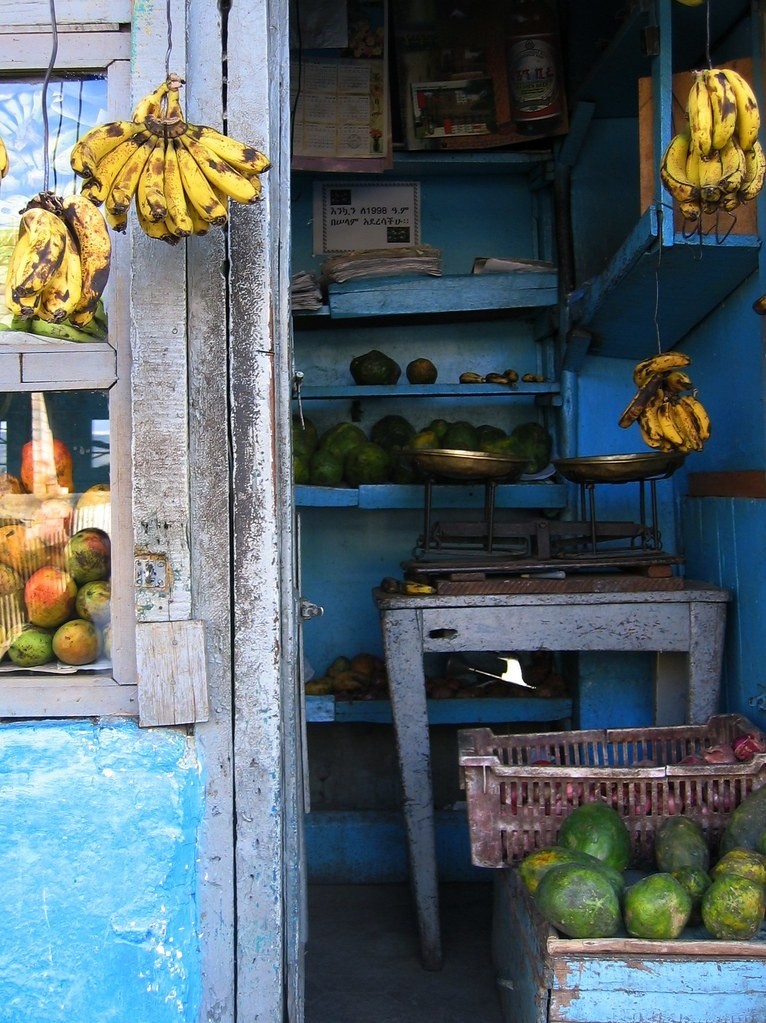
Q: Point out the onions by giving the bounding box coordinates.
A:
[498,732,762,856]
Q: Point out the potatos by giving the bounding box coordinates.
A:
[304,648,571,703]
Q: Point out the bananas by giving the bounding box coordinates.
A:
[71,75,272,247]
[0,136,110,344]
[660,69,766,219]
[618,352,710,454]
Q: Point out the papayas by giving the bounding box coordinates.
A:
[0,439,113,669]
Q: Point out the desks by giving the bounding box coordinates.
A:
[364,571,733,974]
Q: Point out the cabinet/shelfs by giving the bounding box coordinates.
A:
[573,0,766,369]
[286,2,573,877]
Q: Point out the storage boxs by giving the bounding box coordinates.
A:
[456,713,765,870]
